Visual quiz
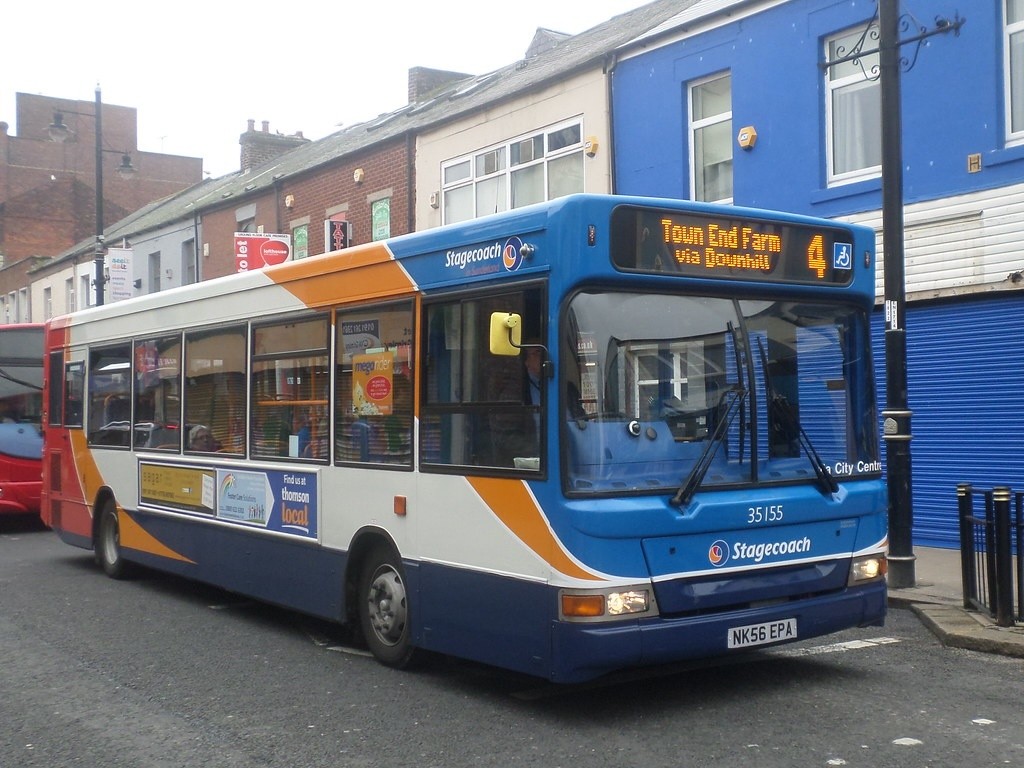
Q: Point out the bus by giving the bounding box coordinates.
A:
[39,188,888,684]
[0,321,50,517]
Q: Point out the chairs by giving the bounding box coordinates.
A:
[351,419,382,464]
[296,427,313,458]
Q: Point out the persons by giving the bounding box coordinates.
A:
[187,424,213,451]
[488,336,586,456]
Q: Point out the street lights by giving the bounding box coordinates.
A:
[44,88,112,306]
